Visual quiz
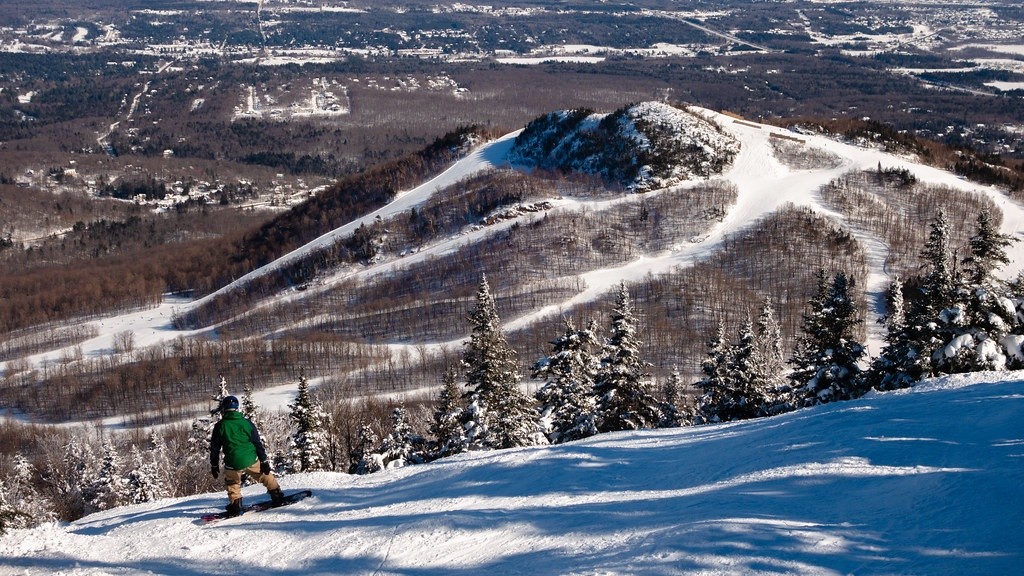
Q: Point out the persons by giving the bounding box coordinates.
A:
[209,396,285,513]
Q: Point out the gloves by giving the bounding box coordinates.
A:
[260,461,270,475]
[211,465,220,479]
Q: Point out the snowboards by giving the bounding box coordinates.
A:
[200,490,312,520]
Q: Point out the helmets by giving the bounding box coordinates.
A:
[221,396,239,409]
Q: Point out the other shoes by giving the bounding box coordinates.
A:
[225,503,243,512]
[272,494,285,504]
